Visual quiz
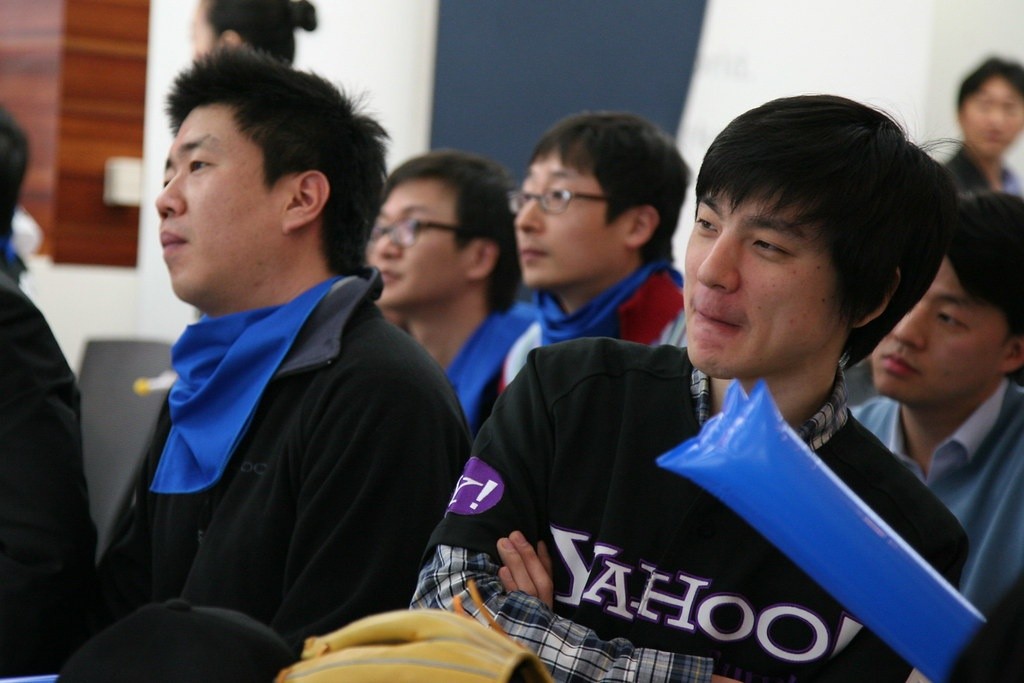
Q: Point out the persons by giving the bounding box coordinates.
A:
[0,0,1024,683]
[494,110,689,407]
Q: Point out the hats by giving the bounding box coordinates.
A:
[50,602,293,682]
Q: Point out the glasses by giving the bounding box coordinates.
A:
[508,188,606,213]
[374,217,456,248]
